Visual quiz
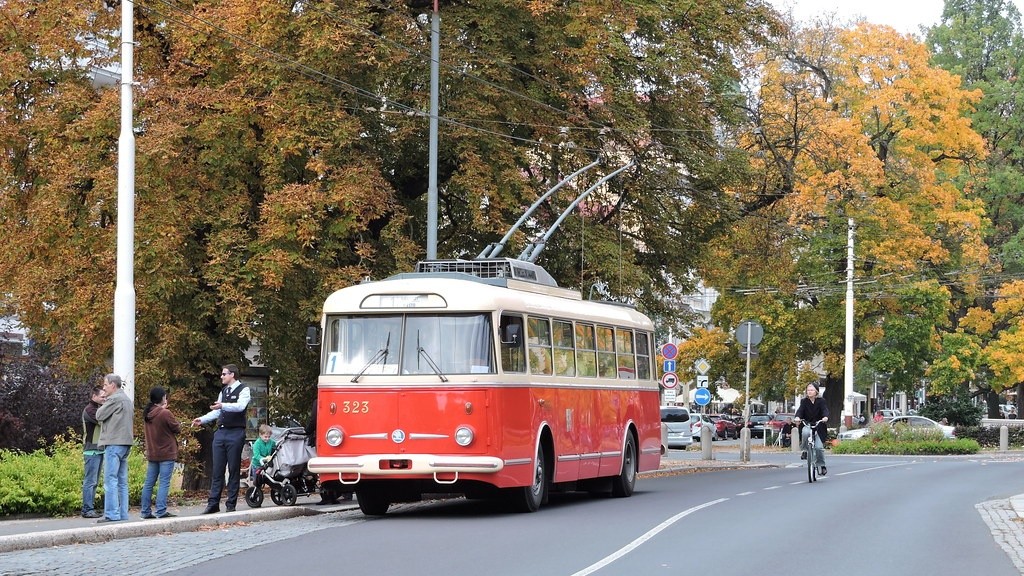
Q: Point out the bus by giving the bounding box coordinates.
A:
[305,258,661,518]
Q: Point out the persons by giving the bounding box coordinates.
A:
[794,383,829,474]
[82,386,106,518]
[782,417,796,448]
[1000,408,1005,419]
[940,418,947,425]
[732,408,737,414]
[873,411,884,425]
[889,413,894,420]
[853,415,865,425]
[775,407,781,414]
[193,365,250,514]
[96,374,133,522]
[1008,407,1017,419]
[350,330,409,374]
[141,387,180,518]
[251,426,276,486]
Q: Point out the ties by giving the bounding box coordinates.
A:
[227,387,232,393]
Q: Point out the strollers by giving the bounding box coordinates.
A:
[245,428,317,508]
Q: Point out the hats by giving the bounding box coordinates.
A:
[806,383,819,394]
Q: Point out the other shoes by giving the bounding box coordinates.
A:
[821,466,827,475]
[94,511,103,517]
[801,452,807,460]
[226,506,236,512]
[202,506,220,514]
[82,510,99,518]
[97,517,120,523]
[141,513,156,519]
[158,512,177,518]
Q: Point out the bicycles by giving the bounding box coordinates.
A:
[793,419,822,482]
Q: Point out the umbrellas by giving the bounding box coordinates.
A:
[675,388,764,415]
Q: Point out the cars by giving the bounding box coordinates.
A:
[874,410,902,422]
[690,413,718,442]
[837,415,956,441]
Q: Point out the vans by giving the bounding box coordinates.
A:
[660,406,693,449]
[999,405,1018,418]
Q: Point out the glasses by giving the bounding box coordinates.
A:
[221,373,231,376]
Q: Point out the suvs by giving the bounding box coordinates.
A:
[708,414,737,440]
[769,413,796,436]
[748,414,774,439]
